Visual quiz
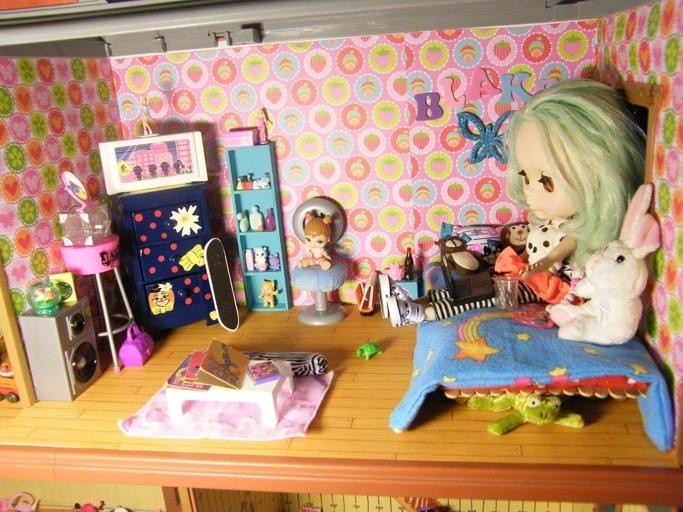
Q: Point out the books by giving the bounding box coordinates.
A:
[167,339,250,391]
[247,361,283,385]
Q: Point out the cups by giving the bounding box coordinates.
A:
[495,277,519,310]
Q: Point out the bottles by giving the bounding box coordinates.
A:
[235,172,276,232]
[244,248,255,271]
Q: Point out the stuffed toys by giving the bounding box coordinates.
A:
[545,183,661,345]
[1,78,674,436]
[467,393,585,435]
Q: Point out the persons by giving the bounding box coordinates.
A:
[295,212,333,271]
[376,79,647,328]
[256,110,269,142]
